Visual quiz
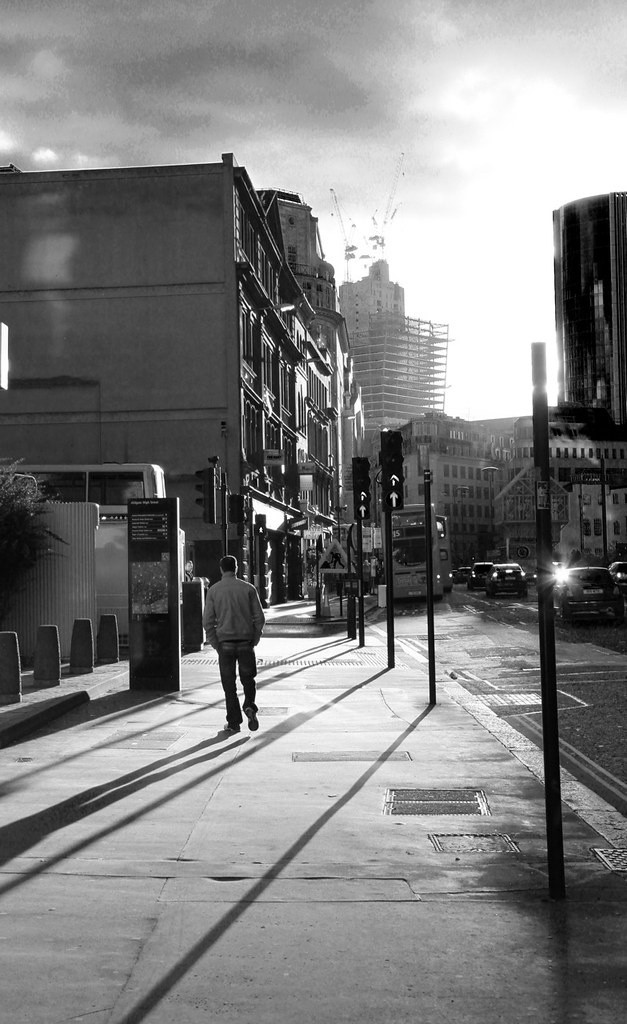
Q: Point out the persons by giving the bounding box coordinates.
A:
[200,555,266,734]
[376,565,383,594]
[362,559,371,596]
[185,559,193,582]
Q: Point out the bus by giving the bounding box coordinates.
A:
[392,504,453,603]
[0,462,167,649]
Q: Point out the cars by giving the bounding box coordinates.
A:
[467,562,494,590]
[486,563,527,599]
[553,567,624,618]
[608,561,627,598]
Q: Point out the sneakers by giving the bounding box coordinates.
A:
[224,723,241,733]
[244,707,260,731]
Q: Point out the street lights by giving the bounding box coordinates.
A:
[482,466,498,561]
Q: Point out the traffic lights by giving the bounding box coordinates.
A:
[195,469,207,508]
[355,459,371,518]
[382,431,404,509]
